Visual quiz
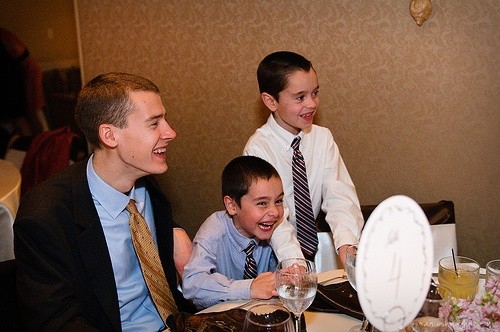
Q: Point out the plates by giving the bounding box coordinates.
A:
[354,195,433,332]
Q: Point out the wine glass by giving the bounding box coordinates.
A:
[275,258,317,332]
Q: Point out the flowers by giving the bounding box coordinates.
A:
[438,275,500,332]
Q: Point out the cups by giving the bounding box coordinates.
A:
[243,304,295,332]
[439,256,480,303]
[485,260,500,299]
[346,243,359,292]
[417,283,452,318]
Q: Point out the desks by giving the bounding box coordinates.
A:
[161,265,486,332]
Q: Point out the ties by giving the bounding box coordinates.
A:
[126,199,179,327]
[243,243,258,279]
[291,136,319,258]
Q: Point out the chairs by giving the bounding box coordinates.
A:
[314,200,458,272]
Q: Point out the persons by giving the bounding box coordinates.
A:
[12,71,199,332]
[243,49,365,275]
[181,155,308,307]
